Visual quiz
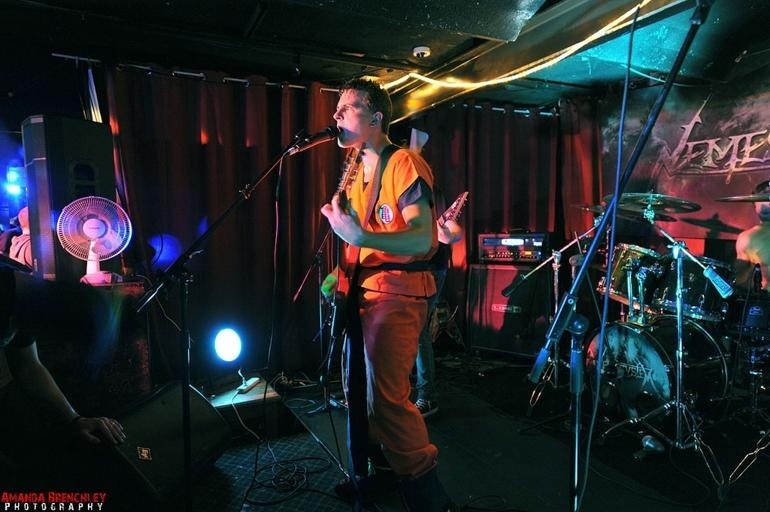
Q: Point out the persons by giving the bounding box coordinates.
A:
[0,215,24,256]
[735,180,769,295]
[1,261,128,447]
[318,79,459,511]
[399,137,465,419]
[7,206,34,271]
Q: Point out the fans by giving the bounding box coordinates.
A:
[54,195,133,286]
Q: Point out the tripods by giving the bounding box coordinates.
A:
[502,226,595,418]
[599,223,734,487]
[293,227,348,415]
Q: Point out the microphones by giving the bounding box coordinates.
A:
[287,126,339,156]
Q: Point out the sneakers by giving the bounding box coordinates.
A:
[414,399,440,418]
[399,468,454,511]
[335,471,399,502]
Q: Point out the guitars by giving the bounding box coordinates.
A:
[435,190,468,228]
[329,141,365,337]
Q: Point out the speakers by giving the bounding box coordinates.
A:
[467,265,572,361]
[21,115,122,281]
[93,382,233,512]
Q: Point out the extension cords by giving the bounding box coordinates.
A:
[237,377,260,393]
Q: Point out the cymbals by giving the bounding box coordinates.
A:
[569,202,672,222]
[714,194,770,203]
[678,217,743,235]
[602,193,702,214]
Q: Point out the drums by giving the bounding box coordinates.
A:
[580,312,728,437]
[607,244,667,307]
[651,253,733,324]
[594,242,629,295]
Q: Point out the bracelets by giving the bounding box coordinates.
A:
[67,415,83,431]
